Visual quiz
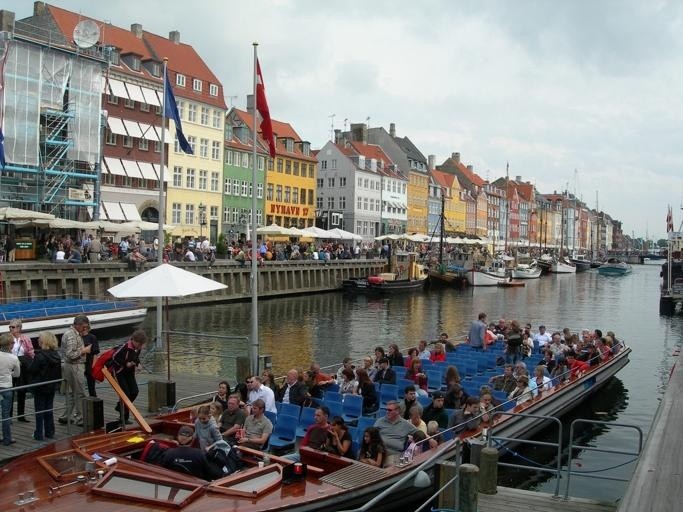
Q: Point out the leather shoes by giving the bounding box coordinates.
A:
[119,418,132,424]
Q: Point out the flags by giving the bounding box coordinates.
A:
[163,63,194,156]
[666,212,672,233]
[255,56,276,159]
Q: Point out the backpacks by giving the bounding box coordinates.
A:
[206,445,242,478]
[91,346,128,381]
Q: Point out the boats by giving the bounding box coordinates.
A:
[341,250,432,296]
[0,322,632,511]
[598,257,633,276]
[0,298,147,350]
[659,205,682,317]
[424,194,467,289]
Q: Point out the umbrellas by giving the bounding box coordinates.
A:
[102,257,229,351]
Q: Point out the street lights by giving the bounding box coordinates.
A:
[199,222,207,242]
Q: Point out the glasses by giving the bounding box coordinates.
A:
[8,325,16,329]
[385,409,391,411]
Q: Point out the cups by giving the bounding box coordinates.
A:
[294,462,303,474]
[104,456,118,466]
[398,455,409,465]
[236,428,245,440]
[258,462,265,468]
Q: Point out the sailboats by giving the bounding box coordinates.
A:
[449,158,602,286]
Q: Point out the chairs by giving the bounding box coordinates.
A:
[0,298,139,322]
[260,335,564,459]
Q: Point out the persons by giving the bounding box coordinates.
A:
[57,316,99,424]
[163,313,624,481]
[8,320,35,423]
[0,333,20,446]
[505,247,584,259]
[226,237,389,268]
[2,228,216,263]
[28,331,62,440]
[395,241,492,262]
[112,328,147,425]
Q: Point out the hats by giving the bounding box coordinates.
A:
[431,391,445,399]
[376,358,388,363]
[178,426,193,437]
